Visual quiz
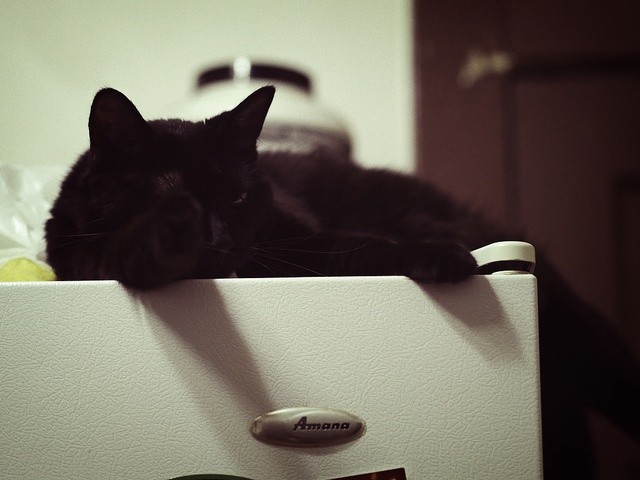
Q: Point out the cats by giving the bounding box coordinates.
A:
[42,81,496,292]
